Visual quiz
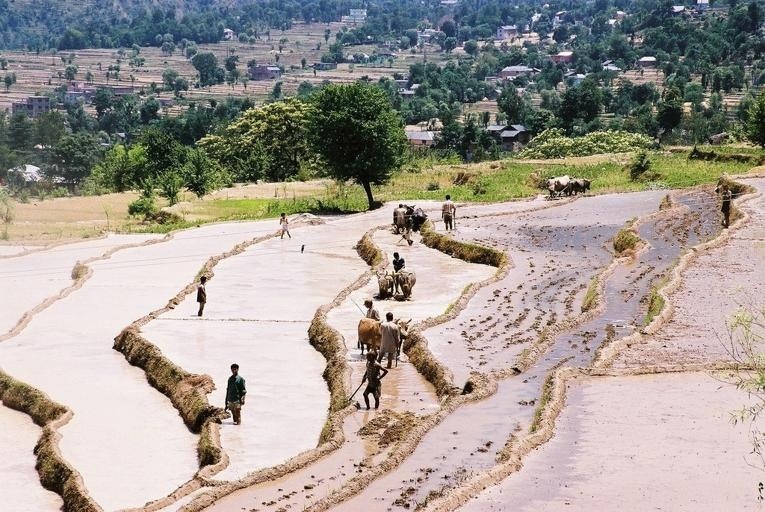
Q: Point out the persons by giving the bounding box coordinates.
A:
[378,312,401,368]
[395,203,405,233]
[393,252,406,292]
[197,276,207,316]
[361,352,388,410]
[442,195,456,230]
[279,213,291,239]
[224,363,247,425]
[403,217,415,245]
[546,175,557,197]
[363,300,380,322]
[721,185,732,227]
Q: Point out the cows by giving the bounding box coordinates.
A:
[393,203,427,231]
[376,270,394,299]
[357,316,413,357]
[552,174,573,196]
[567,177,594,196]
[397,268,417,298]
